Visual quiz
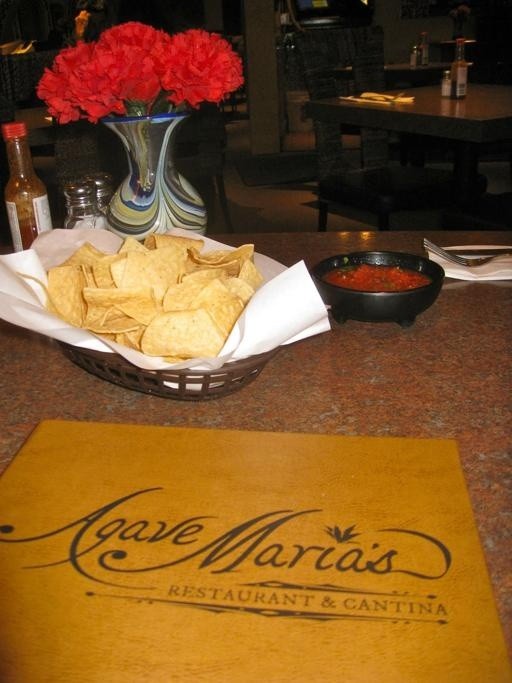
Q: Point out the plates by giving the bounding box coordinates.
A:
[426,243,511,282]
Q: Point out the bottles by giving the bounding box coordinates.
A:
[62,171,115,229]
[451,37,469,100]
[440,71,451,98]
[1,121,53,255]
[408,31,430,67]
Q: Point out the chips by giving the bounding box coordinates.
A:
[46,233,263,364]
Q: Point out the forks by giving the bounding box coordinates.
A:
[421,238,512,267]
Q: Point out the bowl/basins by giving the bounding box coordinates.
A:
[309,249,445,326]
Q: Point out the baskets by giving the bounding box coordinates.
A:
[54,338,283,401]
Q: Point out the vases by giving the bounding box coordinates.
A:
[452,22,464,40]
[102,111,208,246]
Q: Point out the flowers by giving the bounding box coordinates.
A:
[449,4,471,23]
[35,21,246,126]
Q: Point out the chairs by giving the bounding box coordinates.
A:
[291,24,428,232]
[1,48,59,110]
